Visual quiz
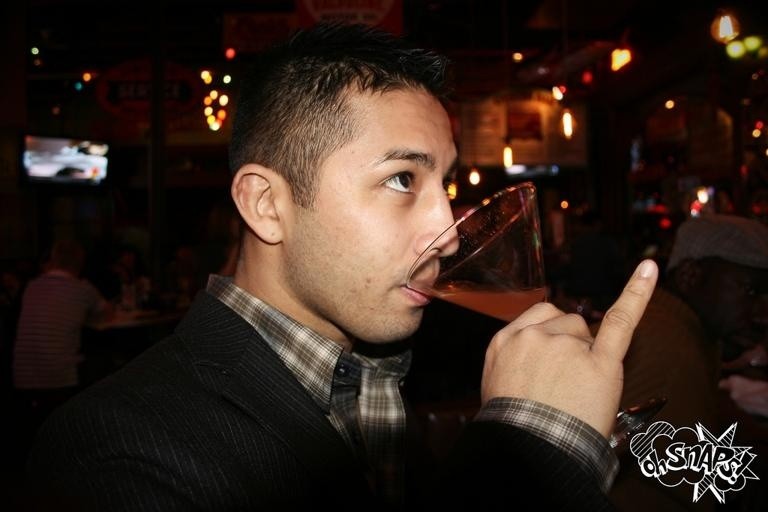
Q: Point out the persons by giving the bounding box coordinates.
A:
[589,213,768,512]
[0,235,199,400]
[24,19,661,511]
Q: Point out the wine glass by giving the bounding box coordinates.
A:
[405,180,668,449]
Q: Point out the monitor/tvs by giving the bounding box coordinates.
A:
[24,135,109,184]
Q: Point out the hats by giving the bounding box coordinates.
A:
[665,213,768,273]
